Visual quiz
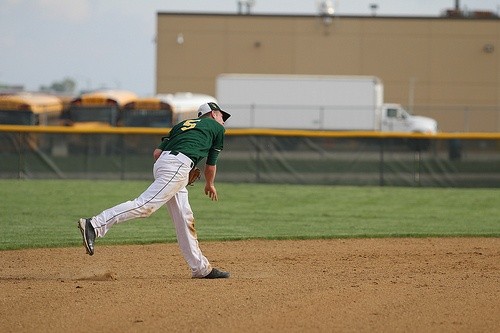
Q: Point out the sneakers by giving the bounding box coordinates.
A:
[193,268,230,279]
[77,218,97,256]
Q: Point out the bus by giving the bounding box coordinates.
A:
[0,88,215,157]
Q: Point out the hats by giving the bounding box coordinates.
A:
[197,101,231,119]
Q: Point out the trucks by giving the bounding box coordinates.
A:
[215,72,438,151]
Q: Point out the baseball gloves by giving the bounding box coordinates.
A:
[189,167,201,185]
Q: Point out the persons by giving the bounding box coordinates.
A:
[77,102,230,278]
